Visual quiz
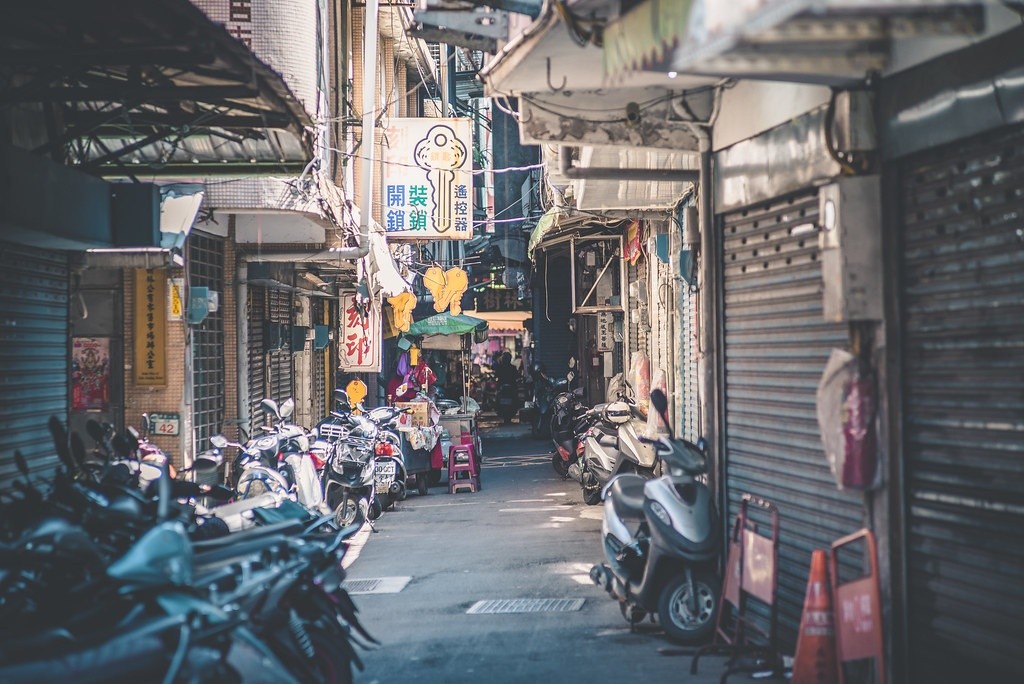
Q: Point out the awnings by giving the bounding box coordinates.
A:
[462,311,533,330]
[1,0,321,184]
[402,309,490,344]
[602,0,983,87]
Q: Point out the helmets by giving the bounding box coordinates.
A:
[602,402,631,423]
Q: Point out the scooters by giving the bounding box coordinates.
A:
[1,383,459,683]
[485,350,730,644]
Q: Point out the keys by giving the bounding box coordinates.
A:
[387,265,469,331]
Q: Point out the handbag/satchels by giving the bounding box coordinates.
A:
[816,347,882,494]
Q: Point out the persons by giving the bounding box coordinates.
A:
[386,366,417,407]
[494,352,518,390]
